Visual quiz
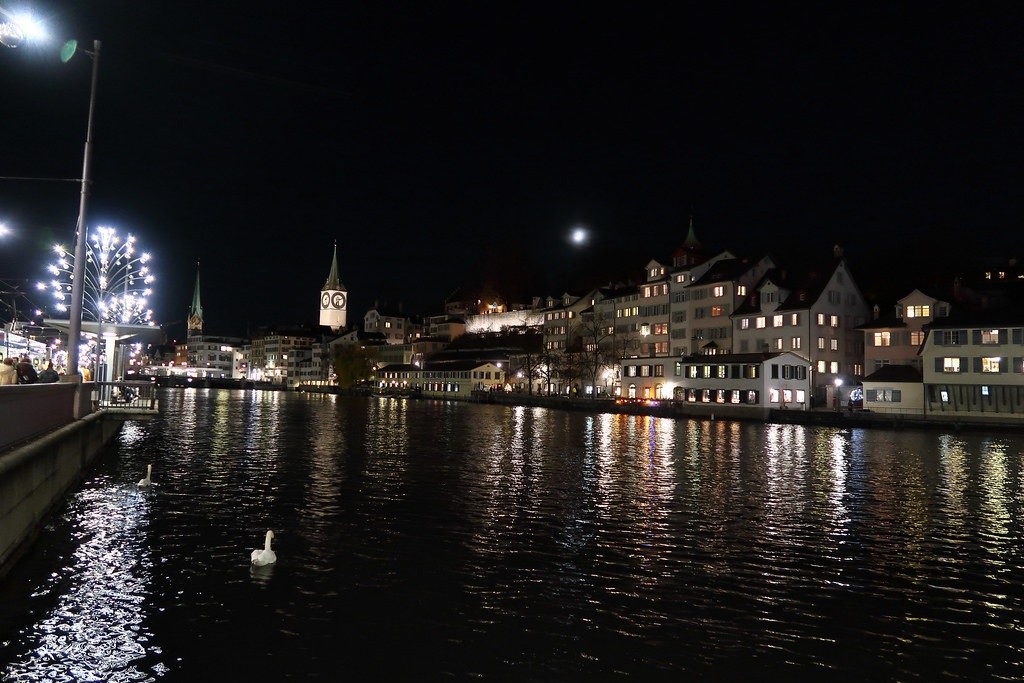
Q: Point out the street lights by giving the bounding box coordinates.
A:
[8,12,101,372]
[592,297,616,396]
[834,376,843,408]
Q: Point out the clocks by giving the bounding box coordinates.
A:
[322,292,331,308]
[331,292,345,309]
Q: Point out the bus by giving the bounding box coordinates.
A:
[0,330,48,363]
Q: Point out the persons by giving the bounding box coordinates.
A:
[16,359,37,384]
[12,357,19,369]
[39,362,60,383]
[0,358,17,385]
[55,363,67,376]
[78,362,96,383]
[114,375,142,399]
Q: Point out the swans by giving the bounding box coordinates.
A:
[250,529,277,567]
[137,464,152,487]
